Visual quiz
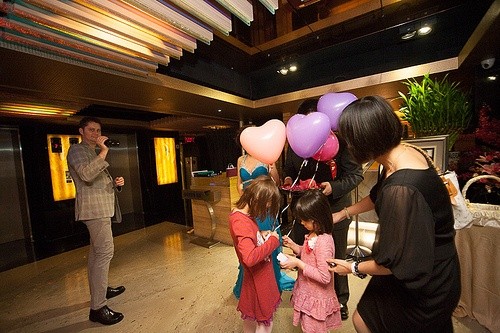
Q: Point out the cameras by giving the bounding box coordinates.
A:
[104,140,115,147]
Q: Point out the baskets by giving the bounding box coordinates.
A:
[462,174,500,228]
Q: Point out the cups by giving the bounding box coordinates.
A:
[226,167,237,177]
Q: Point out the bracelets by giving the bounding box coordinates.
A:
[344,207,350,219]
[351,262,357,276]
[355,259,367,279]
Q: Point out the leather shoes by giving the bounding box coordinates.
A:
[106,285,126,299]
[89,305,124,325]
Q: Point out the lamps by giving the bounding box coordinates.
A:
[399,11,437,40]
[275,54,300,78]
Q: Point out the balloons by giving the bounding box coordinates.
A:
[317,92,358,130]
[287,112,331,159]
[240,119,286,164]
[312,131,339,161]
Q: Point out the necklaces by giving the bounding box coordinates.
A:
[384,147,407,173]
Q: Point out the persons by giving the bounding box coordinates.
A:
[325,95,461,333]
[232,126,296,299]
[465,164,500,205]
[228,175,281,333]
[279,189,342,333]
[67,116,125,325]
[283,99,364,321]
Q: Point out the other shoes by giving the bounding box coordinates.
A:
[339,303,349,318]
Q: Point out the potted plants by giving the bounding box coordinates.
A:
[390,67,473,171]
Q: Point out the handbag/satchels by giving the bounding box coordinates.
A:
[403,141,473,230]
